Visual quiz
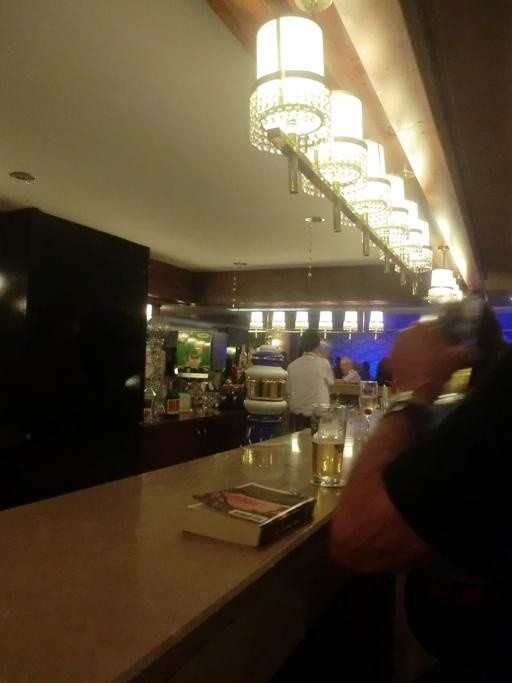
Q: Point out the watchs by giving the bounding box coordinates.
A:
[389,390,432,420]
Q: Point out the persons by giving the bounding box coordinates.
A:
[374,356,389,385]
[321,290,511,682]
[358,361,372,381]
[286,327,332,430]
[335,354,361,410]
[332,356,343,378]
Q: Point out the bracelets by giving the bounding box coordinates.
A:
[381,401,426,431]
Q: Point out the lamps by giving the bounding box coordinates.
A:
[369,310,384,338]
[295,311,309,335]
[249,14,464,303]
[319,310,333,336]
[343,311,358,337]
[272,312,285,337]
[249,312,263,337]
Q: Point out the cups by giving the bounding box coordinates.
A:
[310,404,347,490]
[166,398,181,416]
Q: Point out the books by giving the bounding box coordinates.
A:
[179,478,317,551]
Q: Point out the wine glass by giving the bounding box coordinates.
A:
[359,380,379,441]
